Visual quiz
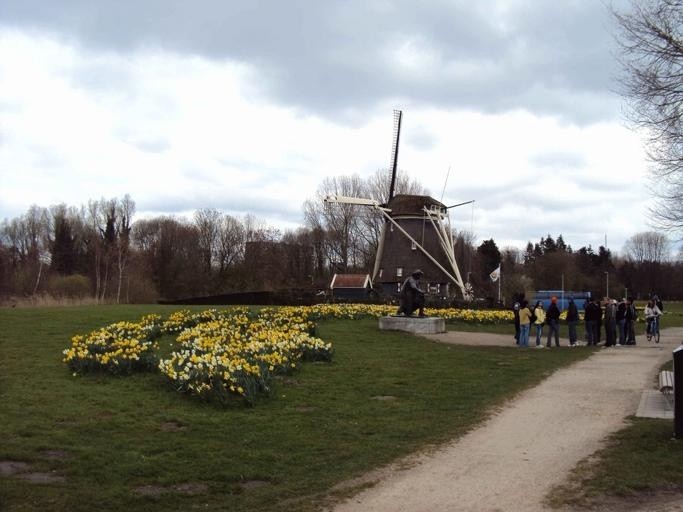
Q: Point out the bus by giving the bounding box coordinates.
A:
[532,289,592,313]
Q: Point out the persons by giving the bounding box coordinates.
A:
[565,296,580,347]
[512,298,522,344]
[396,269,429,318]
[544,295,561,349]
[531,300,546,349]
[516,300,533,347]
[581,293,662,347]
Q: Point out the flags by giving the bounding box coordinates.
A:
[489,266,499,282]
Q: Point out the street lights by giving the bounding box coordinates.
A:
[561,271,565,312]
[604,271,609,297]
[466,271,472,283]
[307,274,314,286]
[624,287,628,300]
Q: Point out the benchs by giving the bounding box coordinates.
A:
[658,370,674,410]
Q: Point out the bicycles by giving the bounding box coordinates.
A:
[645,314,661,343]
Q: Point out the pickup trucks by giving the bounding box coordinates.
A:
[590,297,618,307]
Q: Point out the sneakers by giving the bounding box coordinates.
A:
[515,342,636,350]
[646,335,651,341]
